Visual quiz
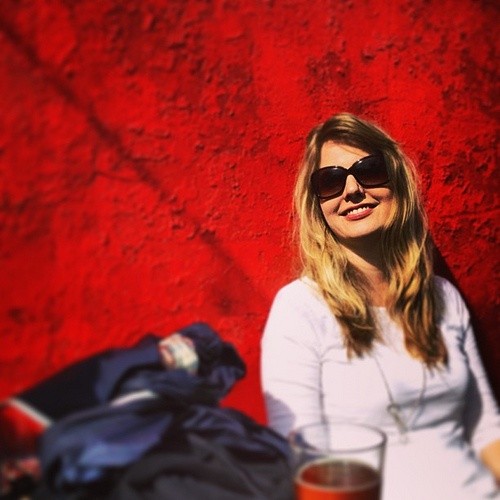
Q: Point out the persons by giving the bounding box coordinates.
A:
[262,112,499,500]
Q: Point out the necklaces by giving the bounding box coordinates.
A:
[369,344,426,437]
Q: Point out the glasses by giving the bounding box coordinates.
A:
[310,155,389,199]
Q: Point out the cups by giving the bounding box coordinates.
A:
[289,421,387,500]
